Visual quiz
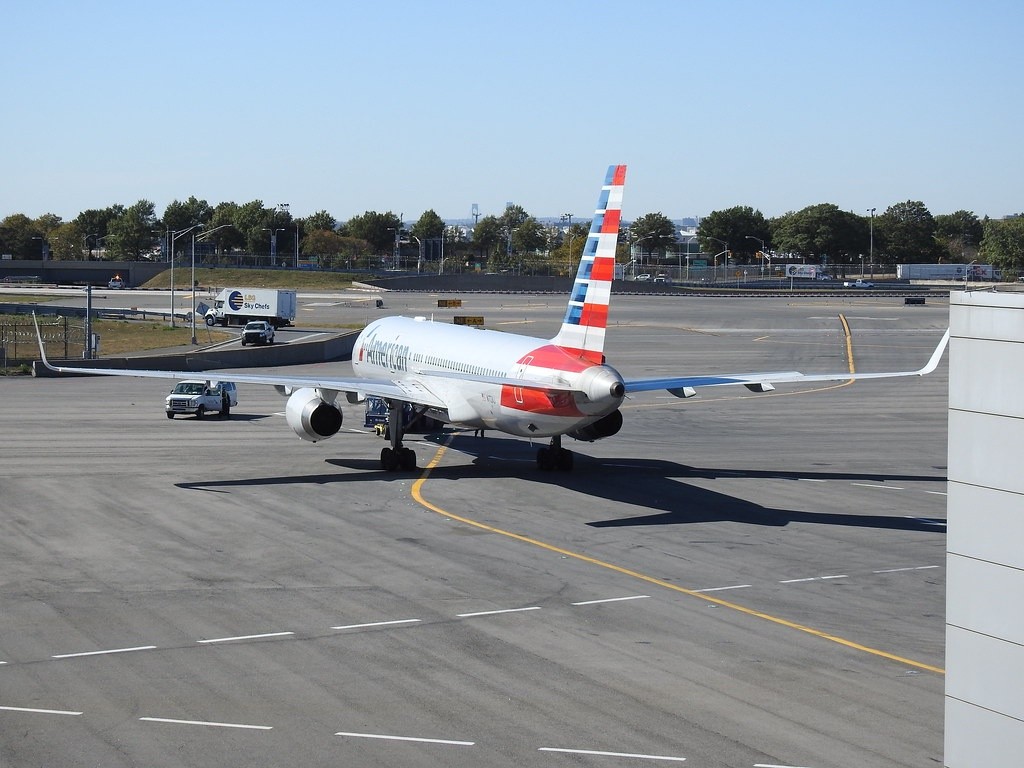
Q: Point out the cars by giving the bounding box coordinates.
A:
[108,278,126,288]
[4,275,41,284]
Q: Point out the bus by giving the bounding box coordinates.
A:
[277,253,331,269]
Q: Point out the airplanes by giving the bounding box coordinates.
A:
[34,165,950,477]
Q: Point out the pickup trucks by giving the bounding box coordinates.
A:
[241,321,275,346]
[843,279,873,289]
[166,380,238,420]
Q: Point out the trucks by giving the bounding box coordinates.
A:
[896,264,1003,282]
[786,264,834,282]
[203,288,297,330]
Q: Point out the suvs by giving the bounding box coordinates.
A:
[654,274,672,283]
[634,274,652,283]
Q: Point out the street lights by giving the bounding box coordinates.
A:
[169,224,204,328]
[192,225,231,344]
[633,231,656,279]
[473,212,482,226]
[387,227,407,269]
[707,237,727,282]
[262,228,285,266]
[32,236,58,261]
[867,207,876,279]
[714,250,730,284]
[83,234,97,260]
[686,236,698,281]
[660,235,682,283]
[565,212,573,277]
[760,251,772,281]
[623,260,636,280]
[745,235,764,278]
[97,235,116,261]
[966,259,977,292]
